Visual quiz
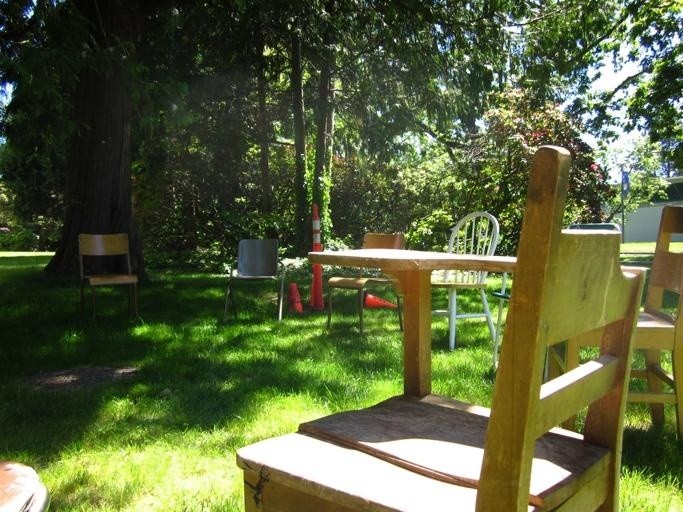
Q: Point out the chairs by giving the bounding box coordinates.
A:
[78,232,140,324]
[432,212,504,349]
[236,145,620,511]
[538,202,683,444]
[224,239,286,323]
[495,222,625,375]
[324,230,408,334]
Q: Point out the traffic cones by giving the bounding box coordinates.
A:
[287,281,304,314]
[363,291,398,309]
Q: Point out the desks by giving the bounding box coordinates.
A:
[313,249,651,429]
